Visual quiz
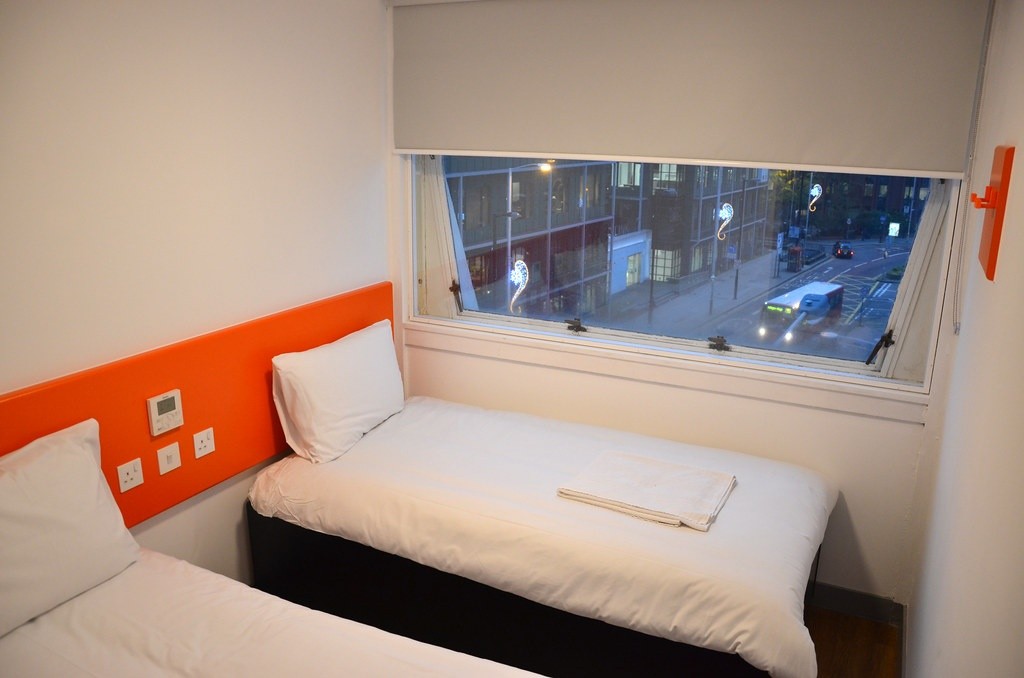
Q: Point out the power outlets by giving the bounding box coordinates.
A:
[117,457,144,494]
[193,427,216,460]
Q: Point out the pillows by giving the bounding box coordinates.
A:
[270,319,404,464]
[0,419,141,640]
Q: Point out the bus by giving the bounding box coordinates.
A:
[757,279,845,338]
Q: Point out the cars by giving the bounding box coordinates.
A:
[832,240,853,258]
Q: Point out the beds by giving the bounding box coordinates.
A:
[0,546,546,678]
[247,391,839,677]
